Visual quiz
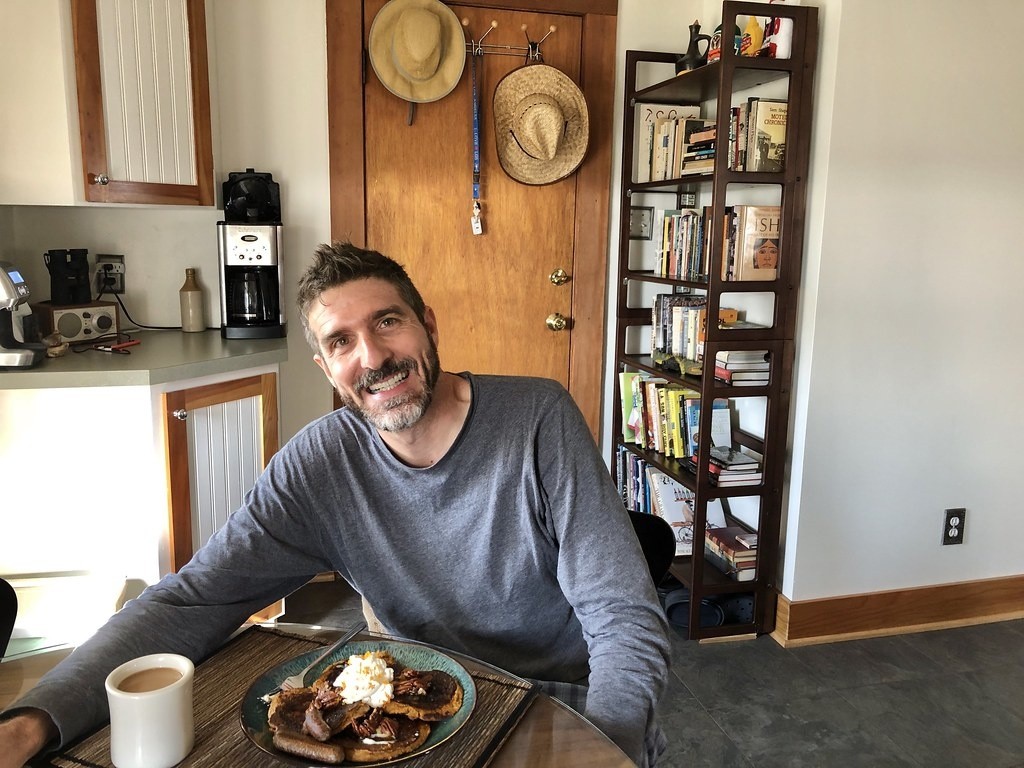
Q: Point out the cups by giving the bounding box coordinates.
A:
[104,653,196,768]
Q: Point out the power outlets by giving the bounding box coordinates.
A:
[942,507,966,546]
[629,206,654,241]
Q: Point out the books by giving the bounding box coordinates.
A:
[615,442,757,583]
[631,95,788,183]
[652,204,782,281]
[648,290,771,387]
[616,369,764,488]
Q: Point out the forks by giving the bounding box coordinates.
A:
[256,621,368,709]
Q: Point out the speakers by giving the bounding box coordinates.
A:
[28,299,120,346]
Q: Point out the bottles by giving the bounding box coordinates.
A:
[179,269,206,333]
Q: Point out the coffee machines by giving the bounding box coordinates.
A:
[215,168,289,340]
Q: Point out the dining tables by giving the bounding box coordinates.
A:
[0,621,638,768]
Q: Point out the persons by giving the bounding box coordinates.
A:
[1,241,678,768]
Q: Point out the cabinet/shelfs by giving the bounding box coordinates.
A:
[70,0,216,206]
[94,253,125,295]
[611,0,819,640]
[161,371,284,628]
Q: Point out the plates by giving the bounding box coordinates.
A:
[238,639,477,768]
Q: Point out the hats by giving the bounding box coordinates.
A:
[369,0,467,104]
[492,63,591,186]
